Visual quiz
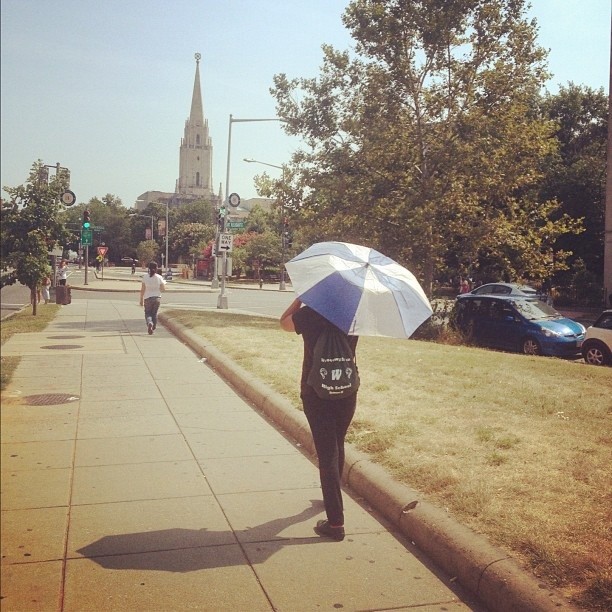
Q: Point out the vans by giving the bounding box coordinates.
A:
[449,294,586,356]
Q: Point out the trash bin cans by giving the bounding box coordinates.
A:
[56,284,71,304]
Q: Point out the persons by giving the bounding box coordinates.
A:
[41,276,52,305]
[459,280,470,293]
[57,259,69,285]
[279,298,360,541]
[139,261,165,335]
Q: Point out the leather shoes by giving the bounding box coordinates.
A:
[314,519,345,540]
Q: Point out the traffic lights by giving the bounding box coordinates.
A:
[83,210,91,230]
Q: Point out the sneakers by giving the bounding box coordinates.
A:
[154,328,157,330]
[148,321,154,334]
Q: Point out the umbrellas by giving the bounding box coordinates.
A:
[283,241,433,340]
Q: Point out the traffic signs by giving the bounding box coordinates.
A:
[219,234,233,253]
[82,228,92,246]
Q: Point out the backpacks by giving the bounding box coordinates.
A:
[307,327,361,401]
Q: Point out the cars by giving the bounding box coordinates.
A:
[579,310,611,366]
[121,257,138,263]
[456,282,552,304]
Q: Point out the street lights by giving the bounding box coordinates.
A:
[134,199,169,281]
[128,213,154,240]
[218,113,291,309]
[241,157,286,290]
